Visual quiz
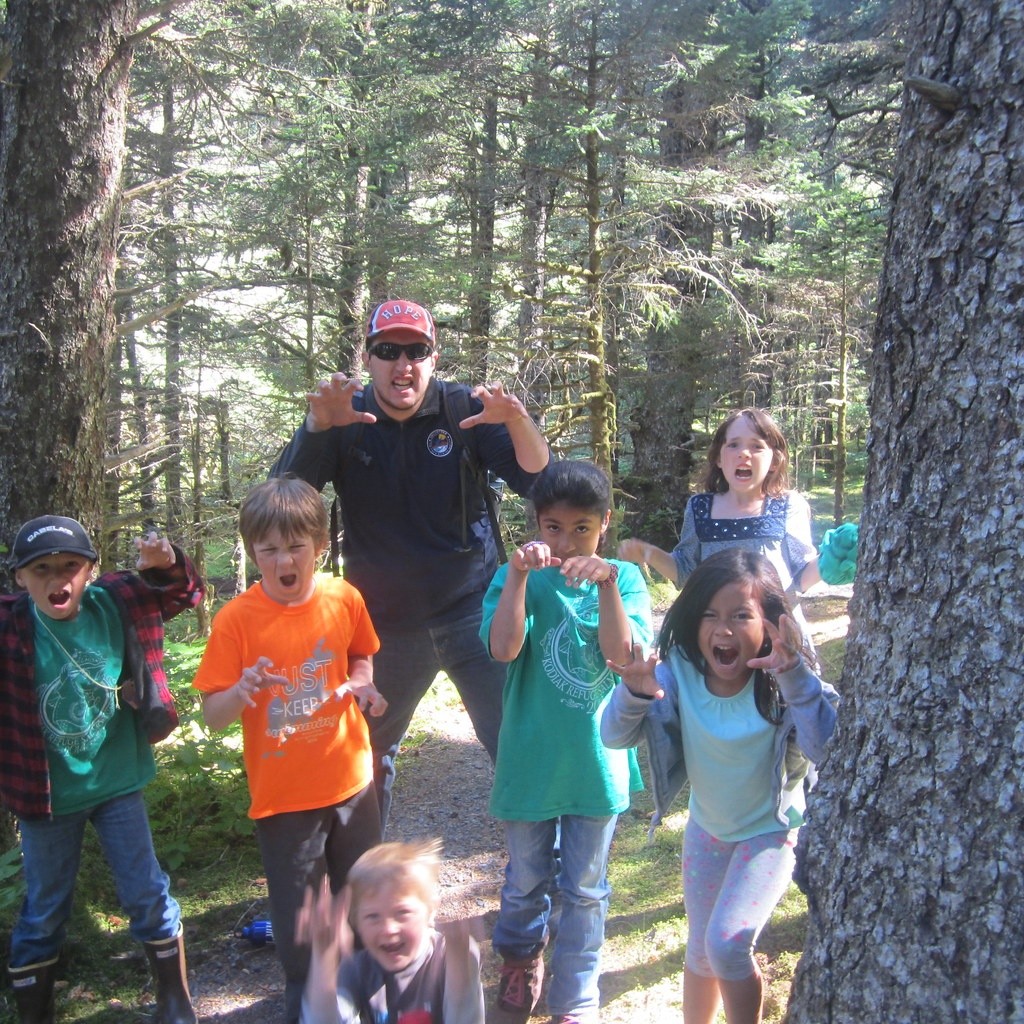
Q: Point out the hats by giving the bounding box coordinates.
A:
[365,299,435,346]
[9,516,98,567]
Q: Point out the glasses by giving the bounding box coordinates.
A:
[366,342,433,362]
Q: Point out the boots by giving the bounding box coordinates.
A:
[8,951,56,1024]
[144,922,197,1024]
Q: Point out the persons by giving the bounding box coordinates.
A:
[190,477,390,1023]
[0,513,204,1023]
[598,546,838,1024]
[617,406,859,609]
[270,300,553,826]
[298,837,486,1023]
[479,461,654,1024]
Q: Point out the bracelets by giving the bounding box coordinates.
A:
[595,560,619,589]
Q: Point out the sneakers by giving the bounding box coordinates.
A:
[497,951,545,1017]
[550,1012,596,1024]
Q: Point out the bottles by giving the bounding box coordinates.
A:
[233,918,275,944]
